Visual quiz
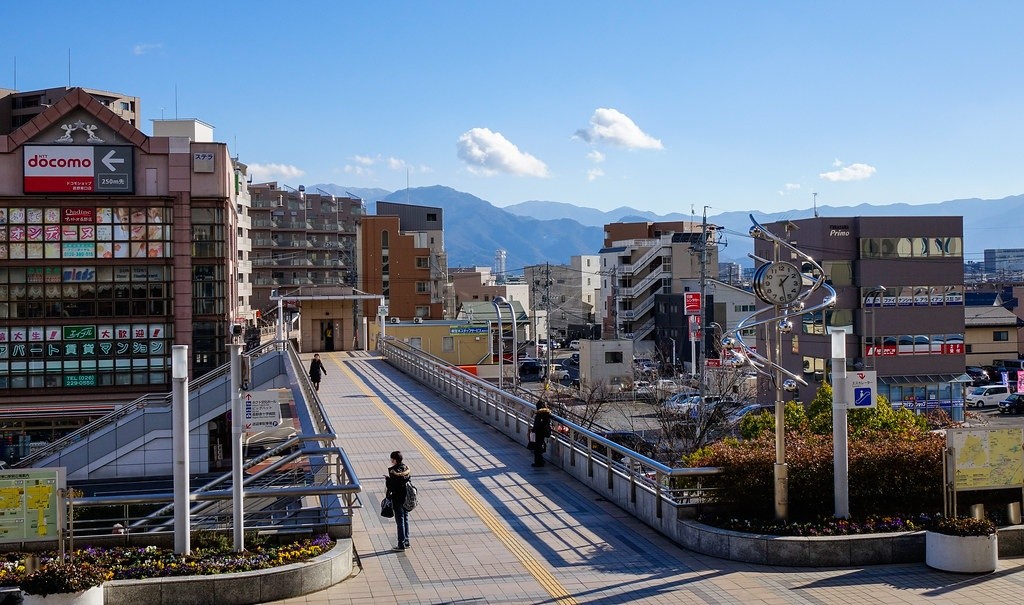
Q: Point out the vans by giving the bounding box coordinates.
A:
[632,357,654,368]
[992,358,1024,380]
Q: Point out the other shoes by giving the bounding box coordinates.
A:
[404,543,410,548]
[531,462,544,467]
[392,547,405,552]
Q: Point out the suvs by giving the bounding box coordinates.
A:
[639,363,657,375]
[582,433,657,460]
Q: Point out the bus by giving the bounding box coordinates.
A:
[518,359,547,383]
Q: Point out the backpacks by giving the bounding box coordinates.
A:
[401,475,418,512]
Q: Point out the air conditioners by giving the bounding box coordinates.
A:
[390,317,400,323]
[413,317,423,324]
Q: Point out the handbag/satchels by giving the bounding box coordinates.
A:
[381,490,395,518]
[527,441,546,453]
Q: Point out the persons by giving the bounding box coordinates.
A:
[61,124,77,139]
[115,207,163,257]
[309,353,327,391]
[531,400,551,467]
[325,325,333,351]
[544,382,550,391]
[519,376,521,386]
[83,125,98,139]
[385,451,411,551]
[112,524,123,534]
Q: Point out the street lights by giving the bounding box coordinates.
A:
[531,287,550,373]
[493,296,516,391]
[669,337,676,375]
[226,323,247,553]
[710,321,724,393]
[588,323,593,340]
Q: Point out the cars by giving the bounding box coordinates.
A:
[997,393,1024,414]
[633,381,656,392]
[965,366,990,385]
[570,353,579,365]
[555,339,568,348]
[538,344,547,354]
[967,385,1011,408]
[651,379,677,389]
[541,365,569,380]
[981,364,1010,380]
[661,392,775,431]
[569,340,580,351]
[539,339,560,350]
